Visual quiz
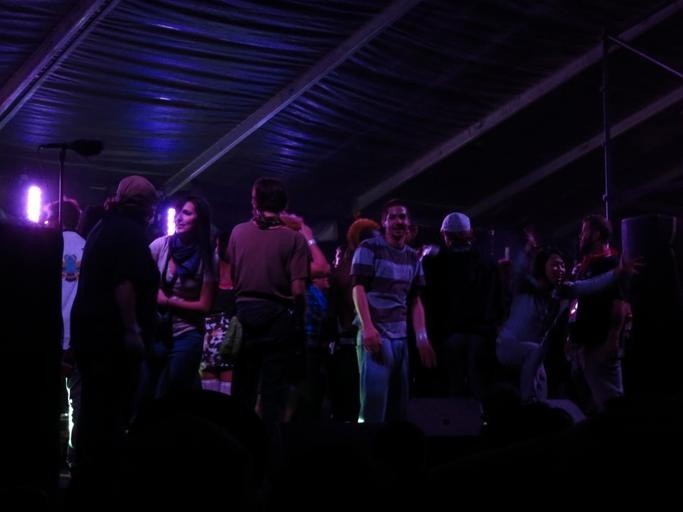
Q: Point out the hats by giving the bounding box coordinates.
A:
[440,212,471,233]
[115,176,161,207]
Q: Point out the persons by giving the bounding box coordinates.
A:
[41,172,649,484]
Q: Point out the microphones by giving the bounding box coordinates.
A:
[38,139,106,158]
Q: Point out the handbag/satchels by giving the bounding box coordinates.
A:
[222,317,243,354]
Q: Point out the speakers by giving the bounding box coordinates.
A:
[621,213,679,269]
